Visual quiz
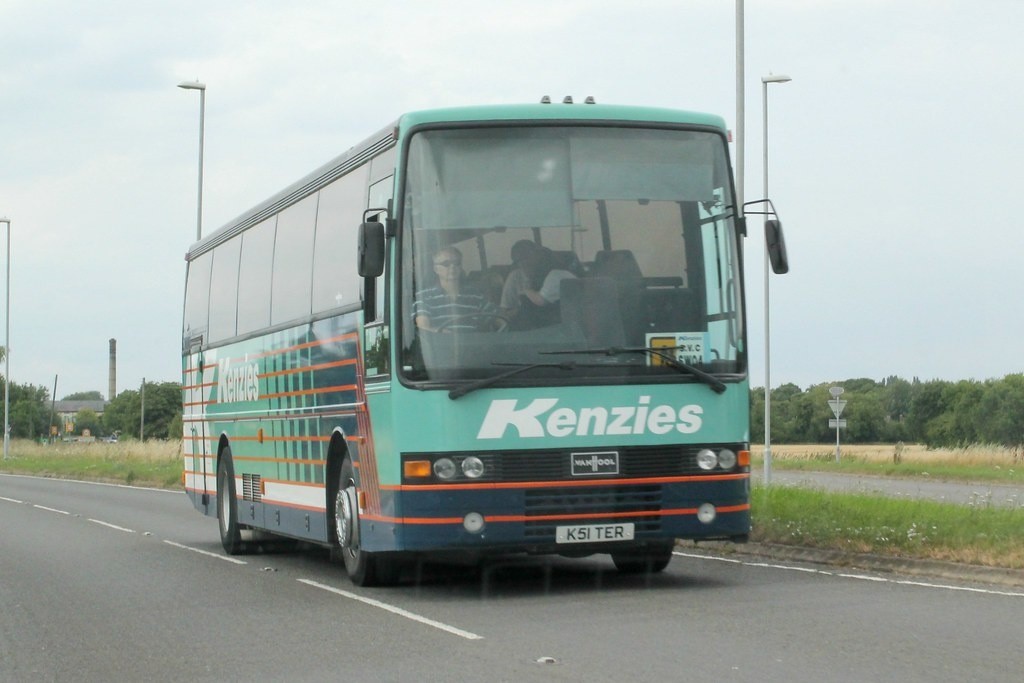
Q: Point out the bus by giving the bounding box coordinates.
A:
[181,93,790,589]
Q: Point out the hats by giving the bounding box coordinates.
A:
[511,239,538,263]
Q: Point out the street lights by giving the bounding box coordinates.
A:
[762,71,794,479]
[0,215,12,461]
[178,79,207,241]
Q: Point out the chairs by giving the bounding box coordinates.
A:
[555,250,584,276]
[593,249,647,293]
[486,264,510,305]
[560,276,626,346]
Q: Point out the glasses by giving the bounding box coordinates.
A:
[433,258,462,268]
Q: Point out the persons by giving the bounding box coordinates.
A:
[411,239,586,333]
